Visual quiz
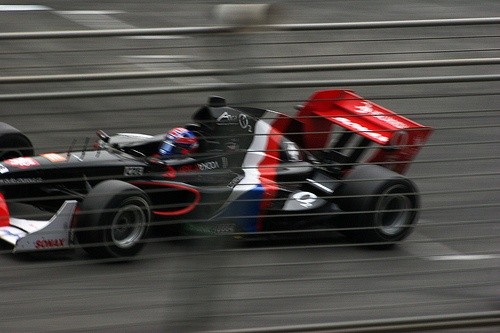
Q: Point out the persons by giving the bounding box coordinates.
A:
[152,128,201,174]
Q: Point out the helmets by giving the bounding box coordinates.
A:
[160,128,199,160]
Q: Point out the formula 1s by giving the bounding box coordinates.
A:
[0,89,433,263]
